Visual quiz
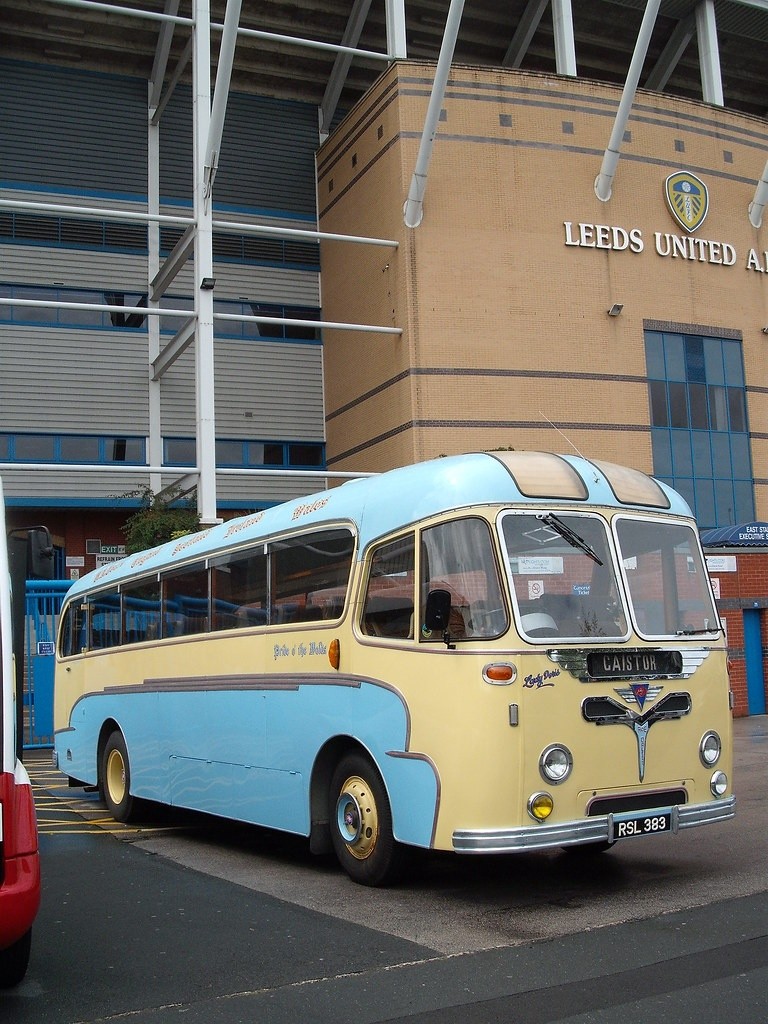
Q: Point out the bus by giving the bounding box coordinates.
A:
[0,475,56,990]
[51,450,737,887]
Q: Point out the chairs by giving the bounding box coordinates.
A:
[148,595,414,639]
